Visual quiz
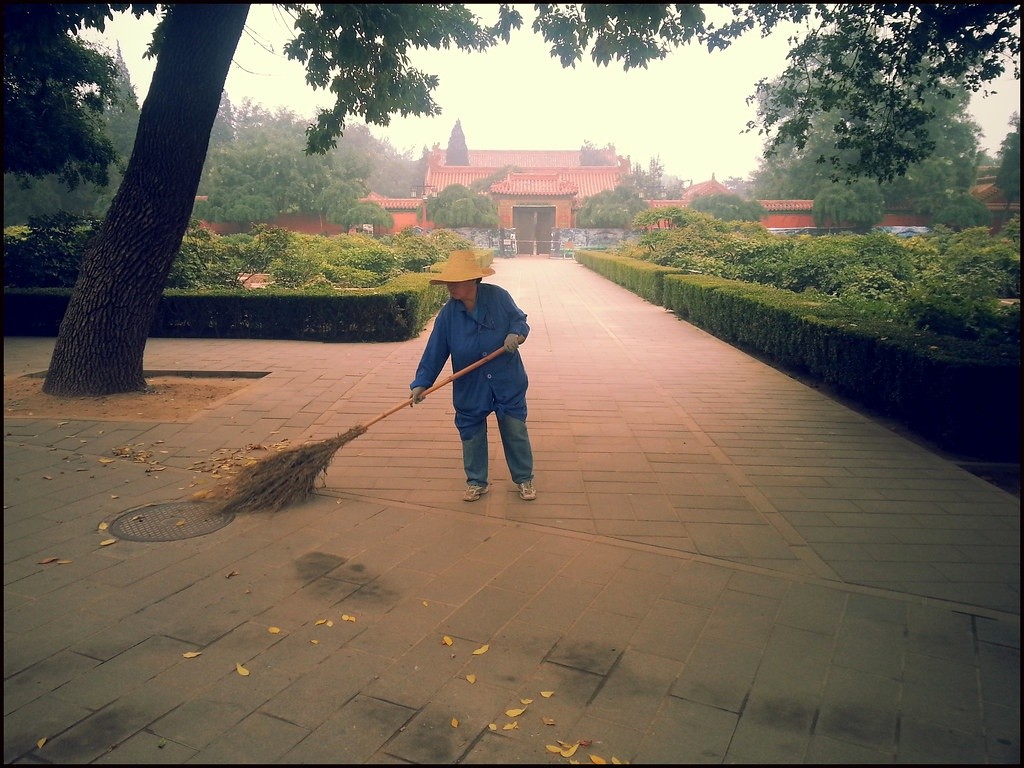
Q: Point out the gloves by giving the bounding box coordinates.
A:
[409,384,426,407]
[504,333,518,353]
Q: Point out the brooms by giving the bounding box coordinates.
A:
[206,333,528,520]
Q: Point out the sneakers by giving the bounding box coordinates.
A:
[517,482,537,500]
[463,481,488,501]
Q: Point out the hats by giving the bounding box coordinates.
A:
[428,249,495,285]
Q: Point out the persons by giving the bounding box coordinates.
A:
[409,249,537,501]
